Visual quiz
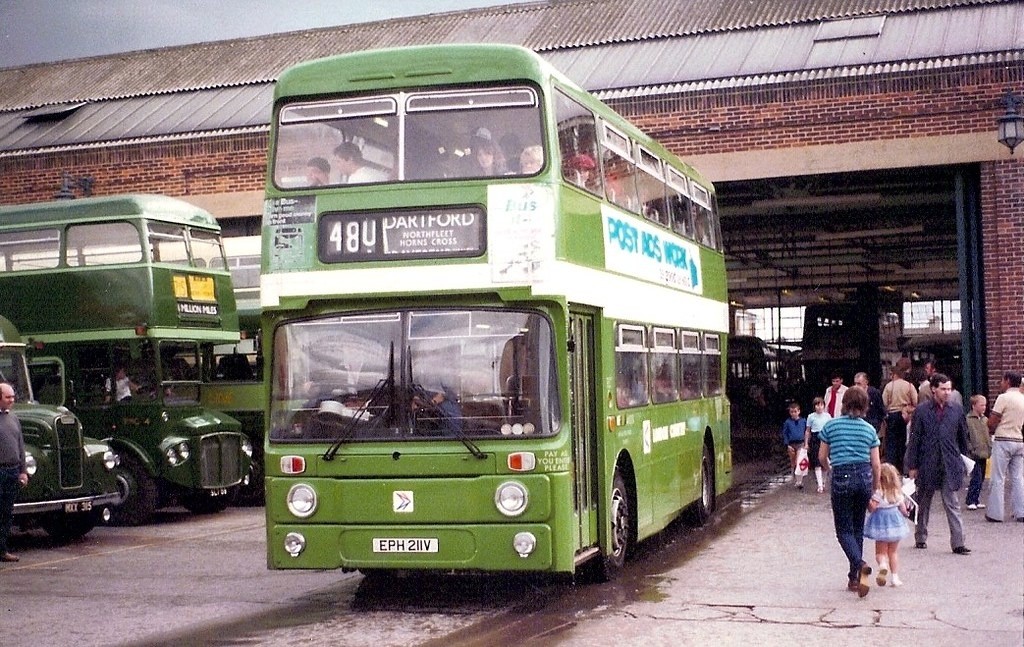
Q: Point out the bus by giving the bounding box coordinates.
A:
[799,307,902,392]
[729,335,779,417]
[1,192,254,517]
[194,308,300,506]
[259,41,731,583]
[1,314,125,545]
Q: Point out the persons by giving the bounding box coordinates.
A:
[0,364,171,562]
[753,357,1024,598]
[292,129,708,439]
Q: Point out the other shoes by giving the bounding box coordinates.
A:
[793,481,804,488]
[848,582,858,589]
[952,546,971,554]
[916,541,927,548]
[1012,515,1024,522]
[817,486,826,493]
[876,568,888,586]
[985,514,1002,522]
[967,504,977,510]
[977,504,985,508]
[856,561,872,597]
[890,580,902,586]
[0,553,19,562]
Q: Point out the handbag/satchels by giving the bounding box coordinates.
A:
[903,443,911,474]
[902,405,915,420]
[795,448,809,476]
[902,476,916,501]
